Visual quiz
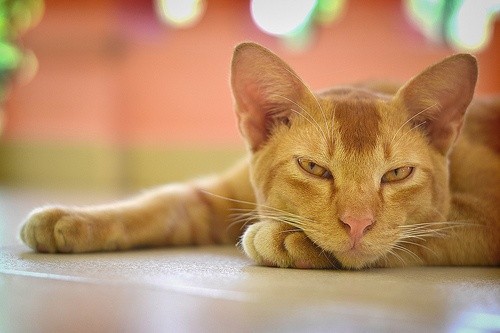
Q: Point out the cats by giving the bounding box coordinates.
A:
[19,41,500,267]
